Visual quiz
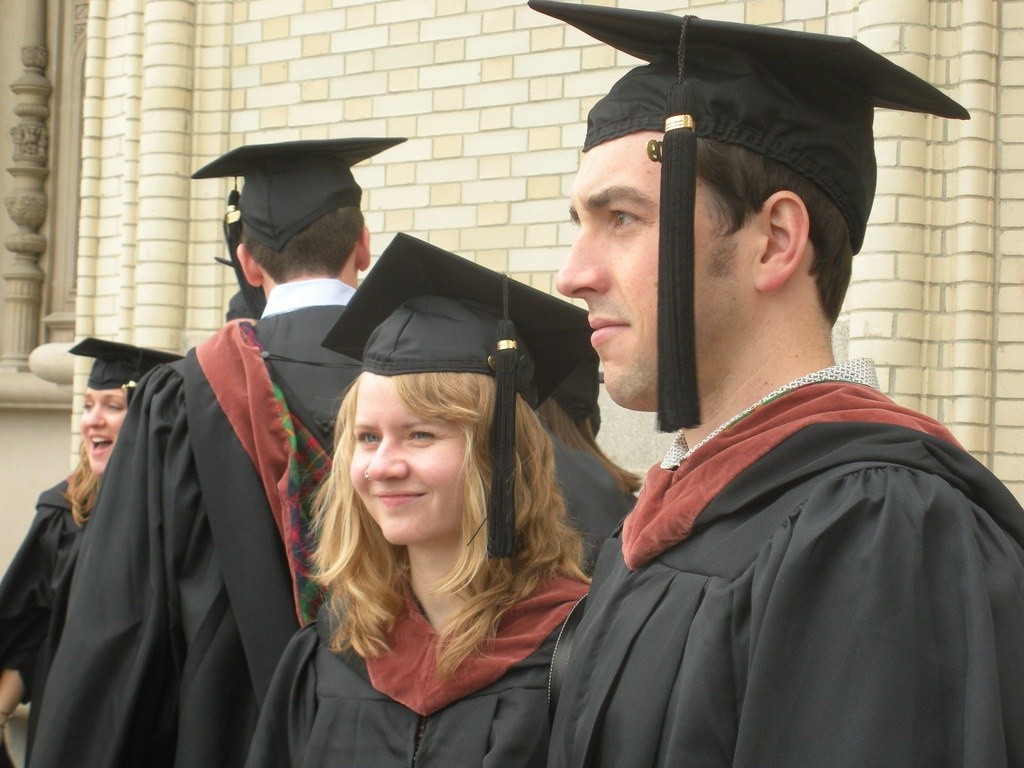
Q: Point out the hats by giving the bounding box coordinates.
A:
[191,136,410,317]
[321,233,599,560]
[68,335,188,407]
[529,1,971,433]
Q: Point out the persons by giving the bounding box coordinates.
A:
[526,0,1024,768]
[0,137,643,768]
